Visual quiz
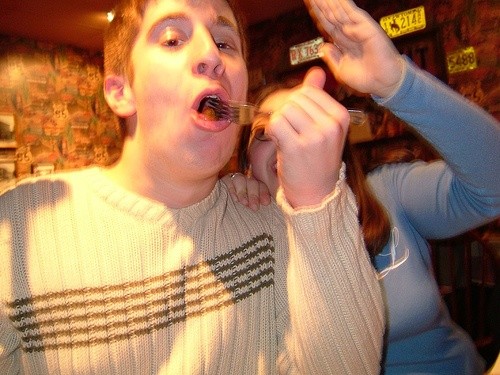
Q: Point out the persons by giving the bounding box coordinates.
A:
[1,0,385,374]
[223,0,500,374]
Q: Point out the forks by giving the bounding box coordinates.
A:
[207,98,366,126]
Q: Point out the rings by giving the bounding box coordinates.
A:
[229,170,242,179]
